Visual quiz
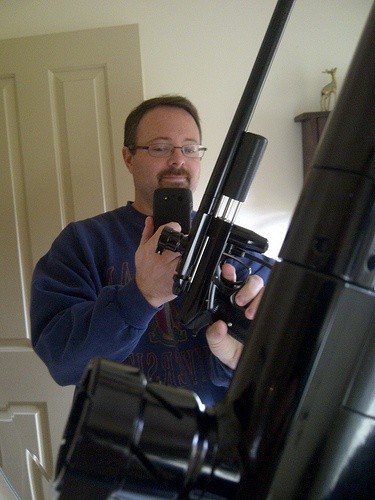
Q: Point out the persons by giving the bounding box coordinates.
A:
[30,96,279,500]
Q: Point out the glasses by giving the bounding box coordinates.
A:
[129,143,207,159]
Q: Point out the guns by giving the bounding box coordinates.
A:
[52,0,375,500]
[153,0,296,345]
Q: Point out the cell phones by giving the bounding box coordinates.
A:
[153,189,194,233]
